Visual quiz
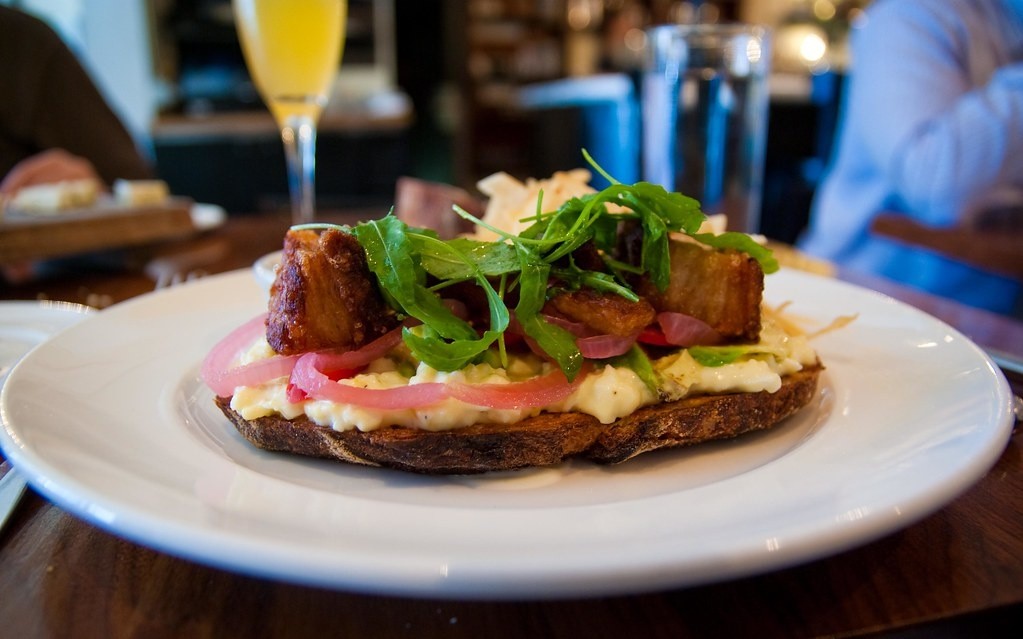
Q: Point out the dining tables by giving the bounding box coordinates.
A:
[0,205,1023,639]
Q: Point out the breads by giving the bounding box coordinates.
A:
[209,366,824,474]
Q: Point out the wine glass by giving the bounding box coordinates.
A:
[230,0,349,256]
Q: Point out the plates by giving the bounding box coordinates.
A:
[1,243,1013,605]
[1,200,228,270]
[0,298,108,426]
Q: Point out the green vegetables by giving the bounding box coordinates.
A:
[286,148,782,381]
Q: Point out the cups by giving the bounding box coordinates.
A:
[642,24,771,243]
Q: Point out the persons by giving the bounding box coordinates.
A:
[0,5,151,280]
[797,0,1023,314]
